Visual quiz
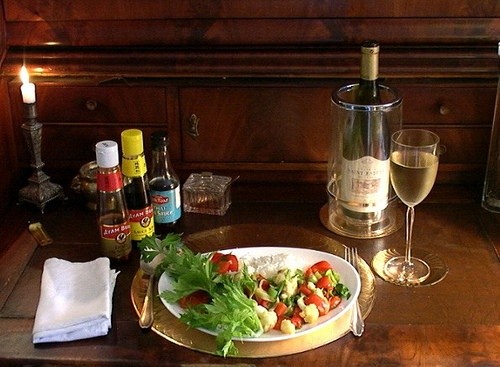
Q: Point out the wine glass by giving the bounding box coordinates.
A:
[382,127,440,284]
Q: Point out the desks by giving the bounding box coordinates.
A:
[0,183,500,367]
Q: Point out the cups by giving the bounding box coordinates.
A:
[324,85,404,238]
[78,161,99,212]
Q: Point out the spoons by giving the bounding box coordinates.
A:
[139,246,163,329]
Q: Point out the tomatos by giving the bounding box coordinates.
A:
[176,253,342,330]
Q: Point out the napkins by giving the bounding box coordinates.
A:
[32,256,121,346]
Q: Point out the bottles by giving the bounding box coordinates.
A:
[341,42,389,223]
[120,128,156,260]
[145,136,183,240]
[95,140,133,266]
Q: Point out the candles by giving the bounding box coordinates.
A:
[20,65,36,103]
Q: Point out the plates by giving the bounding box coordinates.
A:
[158,246,362,343]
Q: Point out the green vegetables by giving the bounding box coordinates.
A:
[250,269,351,315]
[136,232,263,357]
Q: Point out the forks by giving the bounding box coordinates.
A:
[344,247,364,337]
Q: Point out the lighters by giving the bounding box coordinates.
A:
[26,219,53,247]
